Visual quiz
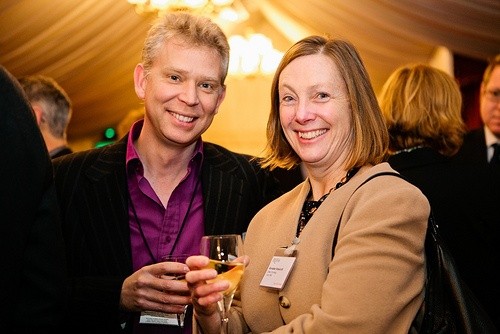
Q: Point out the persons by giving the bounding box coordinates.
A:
[184,36,431,334]
[49,12,296,334]
[1,56,500,334]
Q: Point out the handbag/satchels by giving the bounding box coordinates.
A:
[331,172,471,334]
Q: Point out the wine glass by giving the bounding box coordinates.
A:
[160,256,190,334]
[200,235,245,334]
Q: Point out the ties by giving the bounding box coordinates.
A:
[490,144,500,179]
